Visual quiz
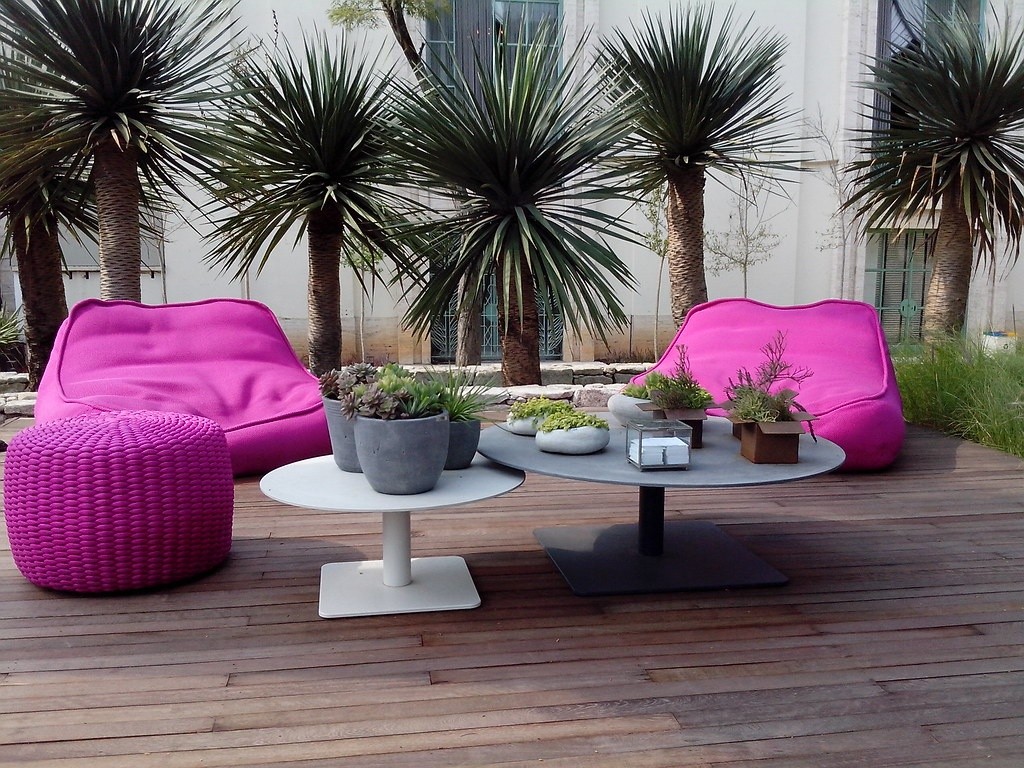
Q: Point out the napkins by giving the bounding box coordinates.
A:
[630,437,690,465]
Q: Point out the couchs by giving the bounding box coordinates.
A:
[630,298,906,469]
[34,299,332,477]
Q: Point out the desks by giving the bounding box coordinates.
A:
[260,450,526,619]
[478,412,847,597]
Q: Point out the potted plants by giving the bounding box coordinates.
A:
[720,329,820,464]
[318,362,449,495]
[636,344,713,448]
[420,357,513,469]
[506,394,610,453]
[608,382,654,427]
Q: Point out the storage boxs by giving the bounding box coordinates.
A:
[625,419,693,471]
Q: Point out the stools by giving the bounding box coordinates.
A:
[3,410,235,594]
